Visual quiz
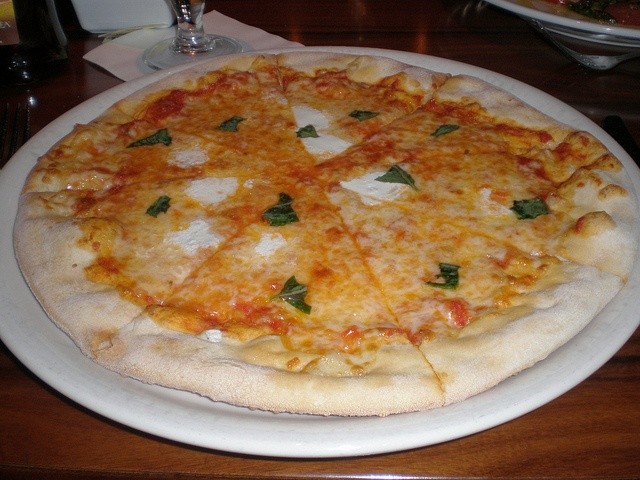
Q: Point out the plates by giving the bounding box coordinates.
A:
[486,0,640,48]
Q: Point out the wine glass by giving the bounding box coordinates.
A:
[144,0,243,75]
